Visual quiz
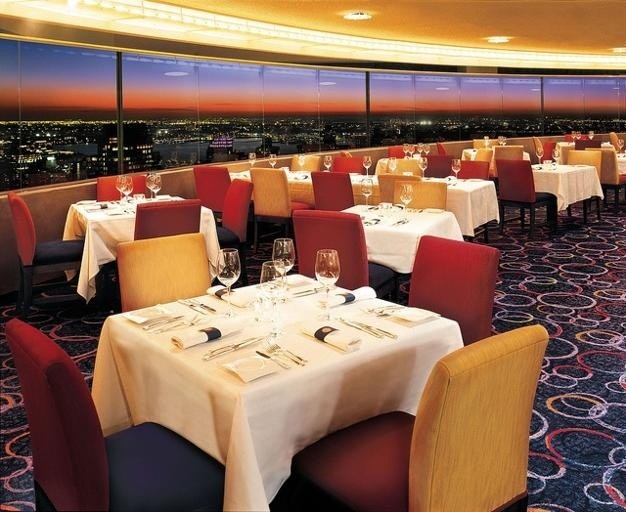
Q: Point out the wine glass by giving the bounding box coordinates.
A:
[498,136,506,146]
[116,173,162,207]
[361,142,461,219]
[617,139,624,157]
[536,148,560,171]
[248,152,334,172]
[571,131,594,145]
[215,237,341,336]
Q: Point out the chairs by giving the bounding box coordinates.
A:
[288,209,398,301]
[290,322,550,511]
[7,191,108,315]
[96,199,201,316]
[217,179,257,286]
[5,315,229,512]
[406,233,501,347]
[193,133,626,245]
[114,232,215,313]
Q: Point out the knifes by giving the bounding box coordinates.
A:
[341,316,398,340]
[256,350,289,369]
[142,298,263,361]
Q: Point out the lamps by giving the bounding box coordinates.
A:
[486,36,509,44]
[613,48,626,53]
[342,12,371,21]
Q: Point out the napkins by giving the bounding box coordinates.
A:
[320,286,377,310]
[171,318,242,350]
[303,322,363,353]
[90,271,468,512]
[206,284,253,309]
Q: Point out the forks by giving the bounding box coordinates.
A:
[262,338,307,366]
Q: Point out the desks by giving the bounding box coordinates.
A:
[59,193,228,303]
[338,203,466,300]
